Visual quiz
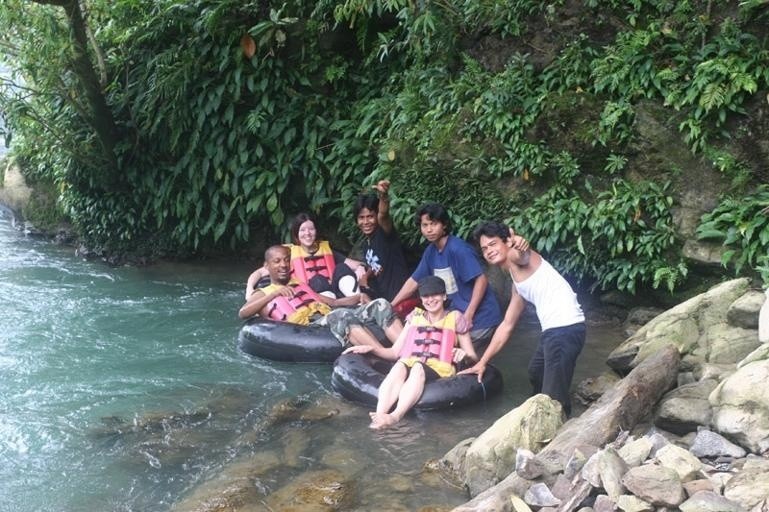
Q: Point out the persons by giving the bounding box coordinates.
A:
[389,203,503,356]
[455,220,586,420]
[352,179,409,303]
[244,213,368,306]
[341,275,478,430]
[238,245,404,361]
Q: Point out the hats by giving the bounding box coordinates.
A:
[418,277,445,296]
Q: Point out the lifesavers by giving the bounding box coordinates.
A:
[330,341,502,414]
[236,250,392,361]
